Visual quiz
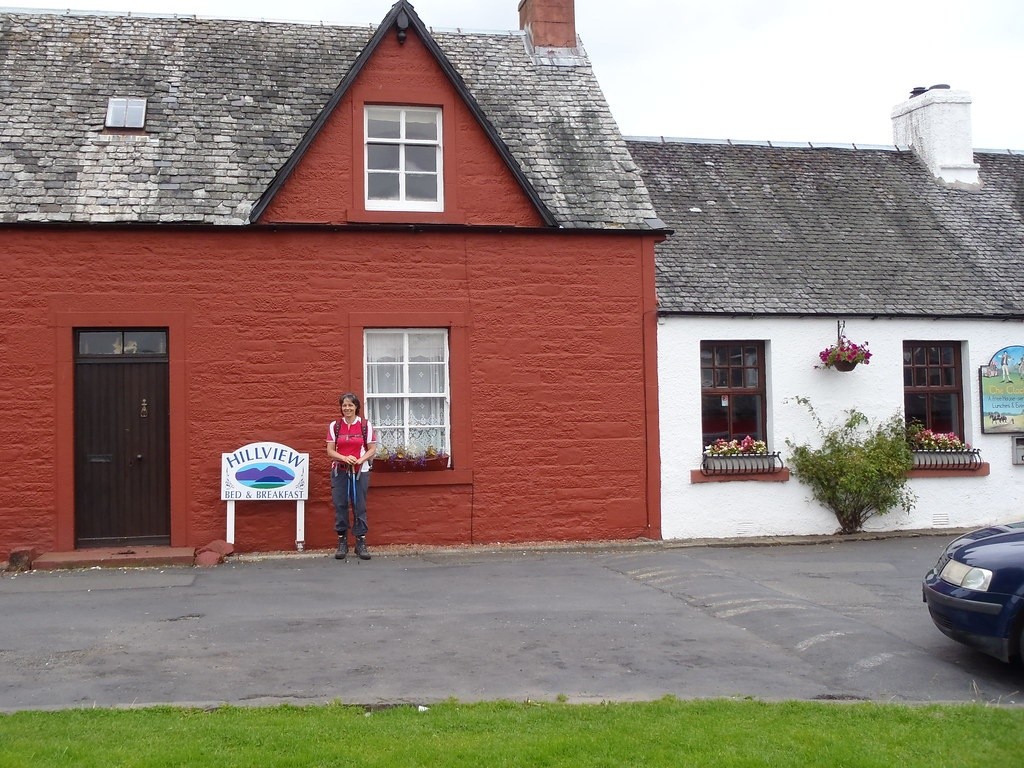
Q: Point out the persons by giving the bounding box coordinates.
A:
[326,392,378,560]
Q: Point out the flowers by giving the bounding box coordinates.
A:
[813,335,874,369]
[380,444,437,461]
[708,435,769,455]
[905,426,969,452]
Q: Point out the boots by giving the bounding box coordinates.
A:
[335,536,348,558]
[354,536,371,559]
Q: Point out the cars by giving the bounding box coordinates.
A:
[921,522,1024,665]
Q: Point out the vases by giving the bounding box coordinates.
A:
[372,453,450,473]
[833,359,857,373]
